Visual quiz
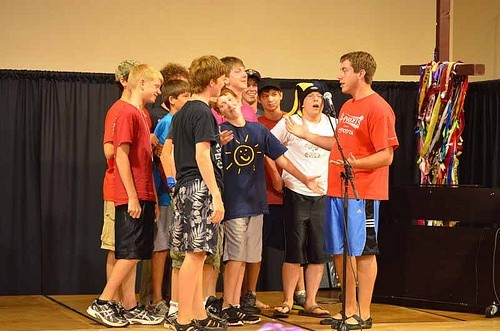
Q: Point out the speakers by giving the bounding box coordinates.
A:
[373,185,500,313]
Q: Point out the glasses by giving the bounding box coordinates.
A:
[306,94,323,99]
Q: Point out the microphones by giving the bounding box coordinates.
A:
[324,92,335,115]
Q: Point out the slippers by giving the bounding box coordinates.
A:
[273,302,292,317]
[298,307,332,318]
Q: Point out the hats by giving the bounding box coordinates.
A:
[245,69,261,82]
[283,82,327,119]
[257,79,282,96]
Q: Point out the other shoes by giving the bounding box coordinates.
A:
[331,315,372,329]
[320,311,351,325]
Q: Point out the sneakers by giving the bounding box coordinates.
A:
[86,300,128,327]
[112,303,123,319]
[205,290,271,326]
[292,291,307,310]
[163,312,178,327]
[171,319,207,331]
[193,314,227,330]
[125,304,164,325]
[144,299,170,317]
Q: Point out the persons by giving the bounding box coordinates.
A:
[286,51,399,330]
[263,83,339,318]
[87,56,307,331]
